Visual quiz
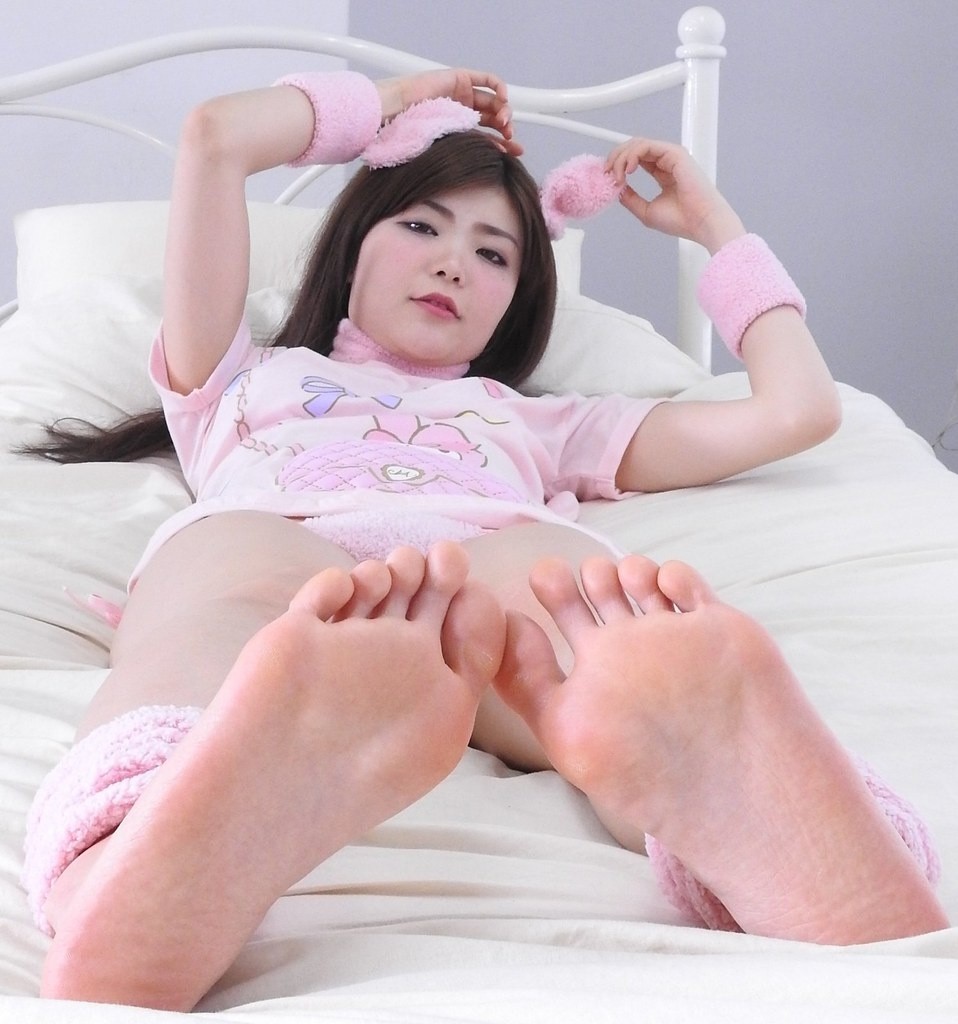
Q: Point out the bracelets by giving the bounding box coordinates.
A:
[696,233,807,362]
[274,68,381,168]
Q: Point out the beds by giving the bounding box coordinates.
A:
[1,2,958,1024]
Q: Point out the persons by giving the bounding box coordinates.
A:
[24,67,951,1012]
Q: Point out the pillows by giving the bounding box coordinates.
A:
[16,200,699,456]
